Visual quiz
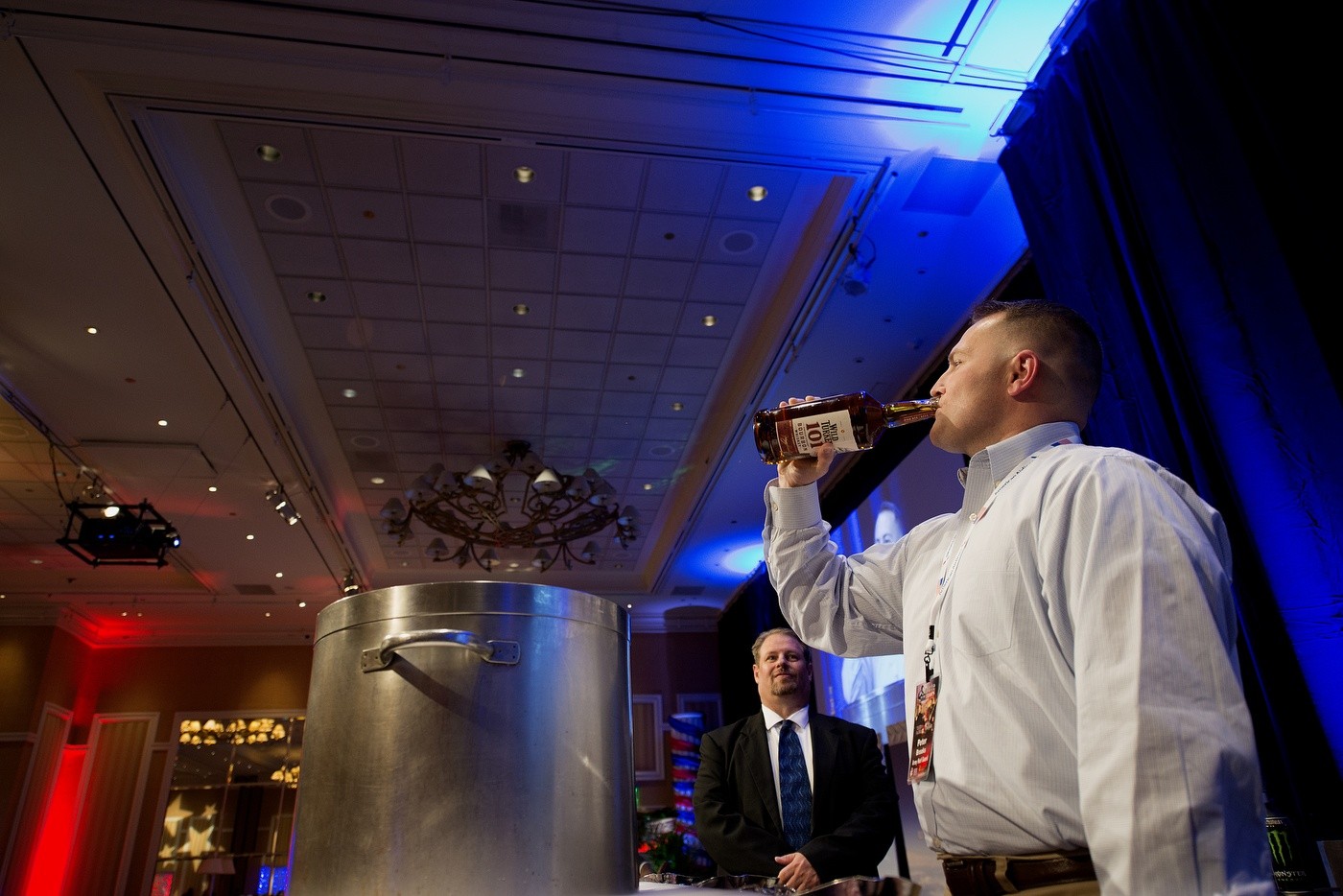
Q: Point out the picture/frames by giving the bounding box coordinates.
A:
[631,693,664,781]
[676,692,724,730]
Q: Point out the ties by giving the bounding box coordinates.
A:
[776,719,814,851]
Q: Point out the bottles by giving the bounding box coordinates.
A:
[753,391,941,465]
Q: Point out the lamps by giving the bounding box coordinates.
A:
[266,491,287,510]
[278,505,298,526]
[343,575,358,592]
[843,266,870,296]
[379,438,645,571]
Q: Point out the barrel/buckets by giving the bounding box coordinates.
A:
[286,580,640,895]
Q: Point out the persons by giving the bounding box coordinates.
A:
[760,297,1281,896]
[691,626,900,896]
[840,501,909,706]
[180,886,286,896]
[910,684,936,775]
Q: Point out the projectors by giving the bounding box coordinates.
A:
[78,519,159,559]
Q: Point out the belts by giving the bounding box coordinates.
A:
[941,848,1099,896]
